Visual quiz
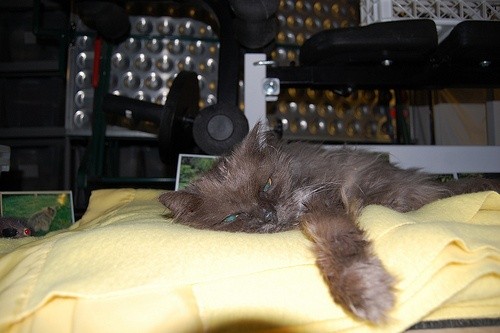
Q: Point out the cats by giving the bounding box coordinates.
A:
[156,119,497,326]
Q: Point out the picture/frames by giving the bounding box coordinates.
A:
[1,190,75,237]
[175,153,224,193]
